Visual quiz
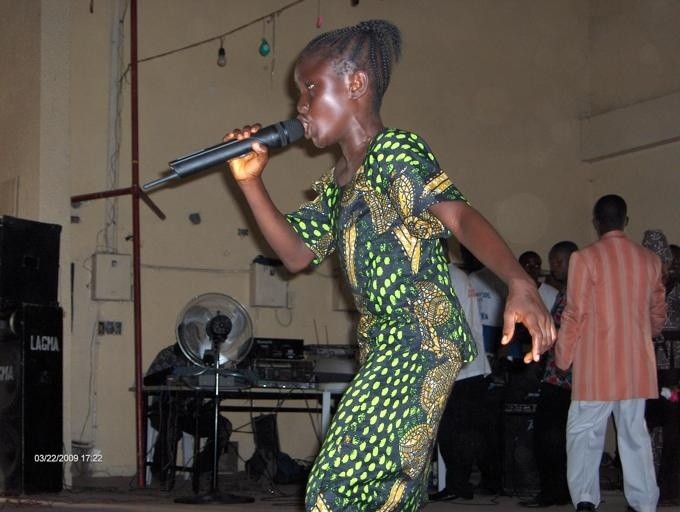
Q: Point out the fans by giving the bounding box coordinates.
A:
[173,291,255,507]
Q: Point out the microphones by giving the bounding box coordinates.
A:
[143,117,306,190]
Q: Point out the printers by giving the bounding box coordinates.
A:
[304,344,360,383]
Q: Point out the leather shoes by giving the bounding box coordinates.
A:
[428,487,474,500]
[519,494,568,508]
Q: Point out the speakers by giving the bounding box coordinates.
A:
[0,214,65,498]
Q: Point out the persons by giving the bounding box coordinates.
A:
[223,20,558,512]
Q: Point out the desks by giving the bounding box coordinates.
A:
[124,375,332,500]
